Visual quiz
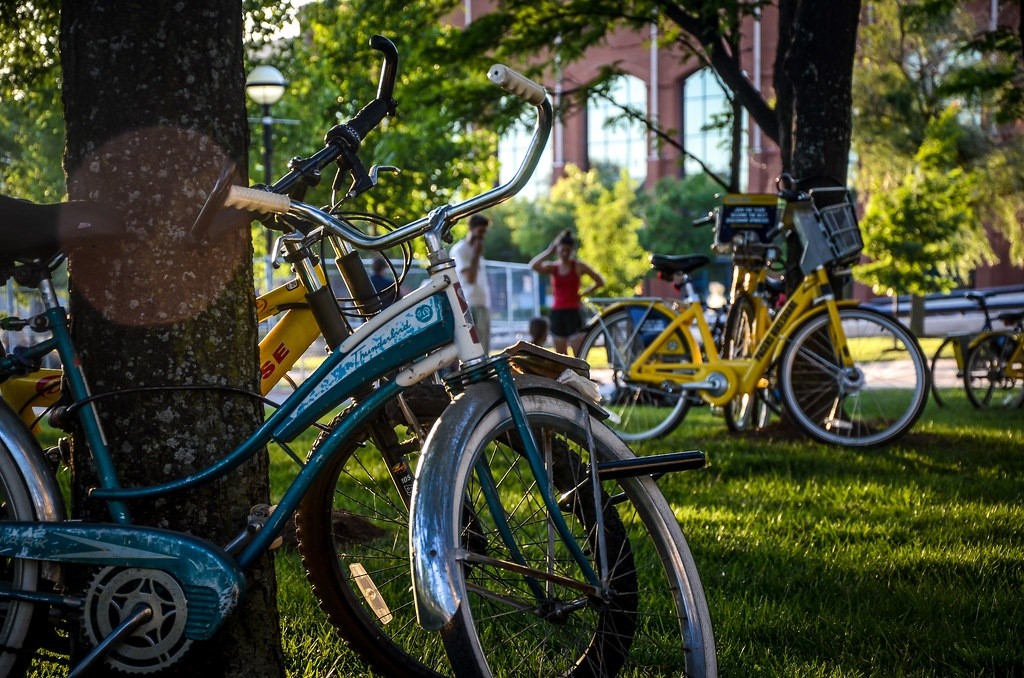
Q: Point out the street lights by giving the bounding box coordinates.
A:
[245,65,289,331]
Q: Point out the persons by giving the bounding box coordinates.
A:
[448,214,485,354]
[528,318,548,346]
[368,257,399,308]
[530,227,604,358]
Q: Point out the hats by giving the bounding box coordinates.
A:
[373,257,389,268]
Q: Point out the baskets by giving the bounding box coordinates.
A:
[808,187,864,271]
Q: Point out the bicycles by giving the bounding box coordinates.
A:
[575,172,930,450]
[930,291,1024,412]
[0,34,718,678]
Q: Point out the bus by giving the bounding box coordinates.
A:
[252,258,538,346]
